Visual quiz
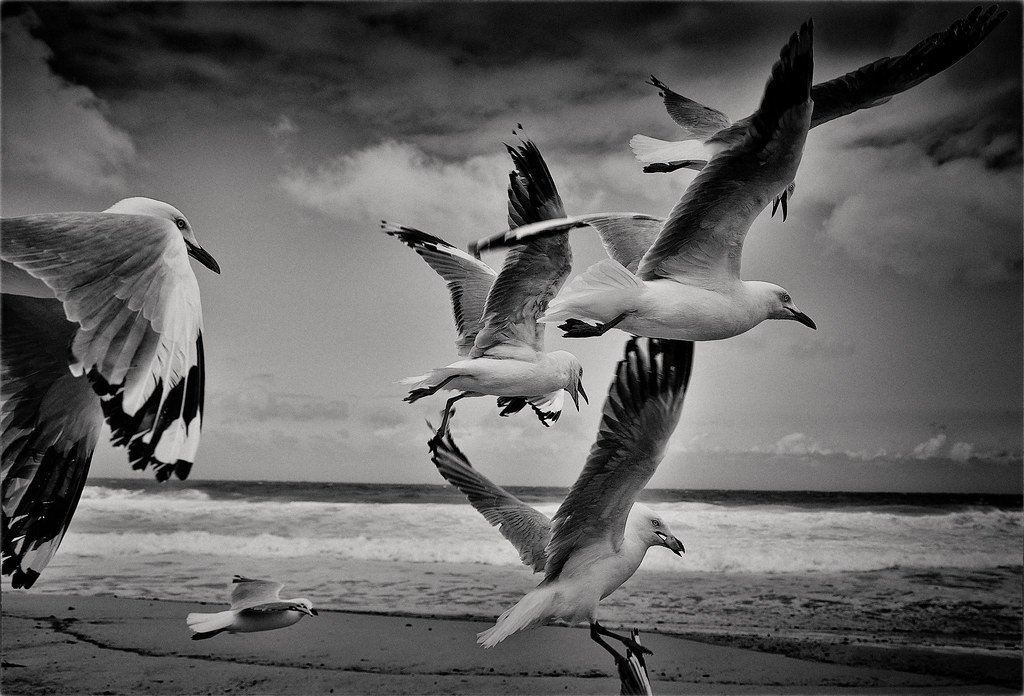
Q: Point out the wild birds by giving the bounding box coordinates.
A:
[468,18,820,338]
[430,336,693,680]
[0,196,223,587]
[377,122,591,454]
[178,565,319,641]
[627,4,1012,223]
